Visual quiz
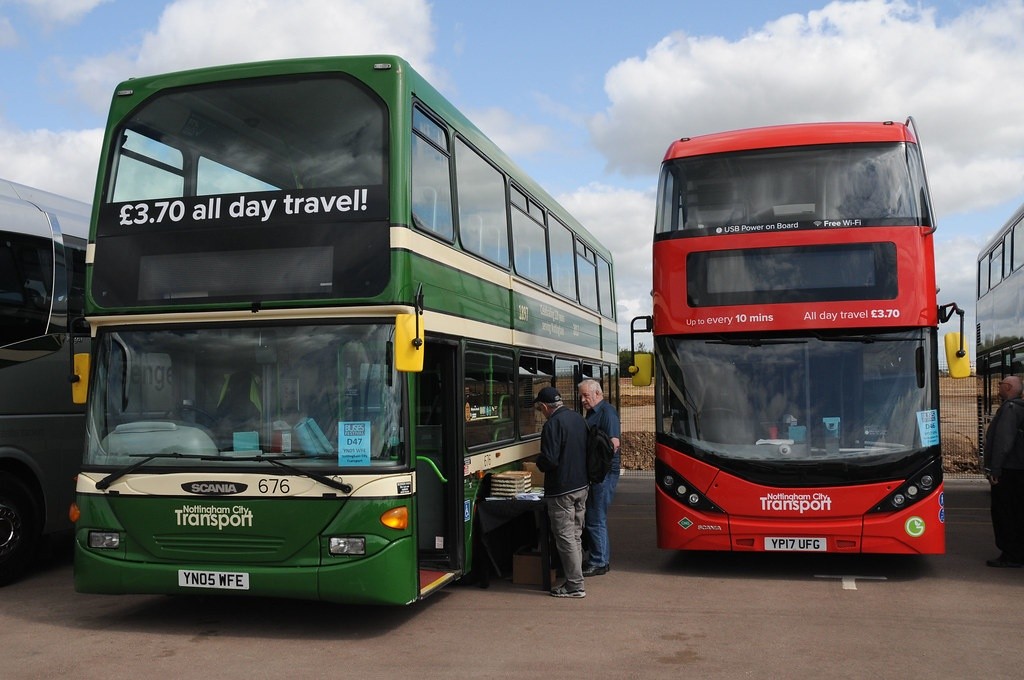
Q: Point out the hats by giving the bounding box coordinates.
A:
[533,387,561,403]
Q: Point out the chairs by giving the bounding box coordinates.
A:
[414,188,593,306]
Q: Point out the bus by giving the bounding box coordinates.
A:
[1,180,177,588]
[629,115,970,554]
[975,204,1024,484]
[73,56,620,608]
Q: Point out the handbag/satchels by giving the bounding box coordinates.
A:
[583,418,614,486]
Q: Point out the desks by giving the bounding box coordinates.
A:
[477,486,555,590]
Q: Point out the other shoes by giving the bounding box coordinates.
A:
[583,560,610,572]
[583,568,605,577]
[987,557,1022,567]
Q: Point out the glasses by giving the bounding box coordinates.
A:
[999,381,1012,388]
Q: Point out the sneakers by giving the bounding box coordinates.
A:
[551,584,586,598]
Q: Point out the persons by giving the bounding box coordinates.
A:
[533,388,590,598]
[984,376,1024,568]
[769,367,825,420]
[578,380,621,577]
[219,349,300,445]
[306,343,387,458]
